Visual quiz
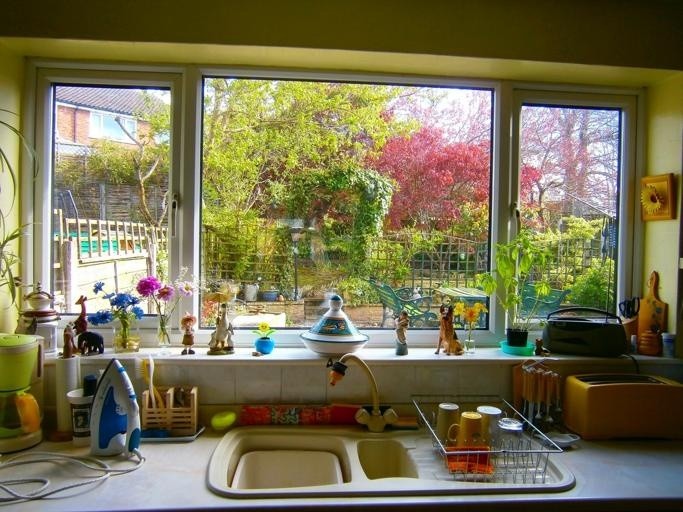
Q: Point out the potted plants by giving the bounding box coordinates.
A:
[259,283,280,302]
[469,225,558,346]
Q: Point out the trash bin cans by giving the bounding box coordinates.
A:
[244,284,259,302]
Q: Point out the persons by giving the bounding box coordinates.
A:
[179,312,196,355]
[394,311,410,345]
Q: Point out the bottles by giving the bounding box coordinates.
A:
[631,335,638,355]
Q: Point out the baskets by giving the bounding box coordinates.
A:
[409,394,563,483]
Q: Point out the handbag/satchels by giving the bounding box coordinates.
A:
[542,307,626,357]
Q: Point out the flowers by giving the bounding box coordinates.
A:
[639,184,665,216]
[86,265,209,346]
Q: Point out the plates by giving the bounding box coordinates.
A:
[533,432,580,448]
[139,426,205,442]
[498,340,535,356]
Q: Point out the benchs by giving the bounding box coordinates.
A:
[367,278,439,327]
[515,283,571,320]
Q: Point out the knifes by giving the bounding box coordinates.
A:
[619,296,640,319]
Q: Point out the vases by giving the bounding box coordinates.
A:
[111,320,142,353]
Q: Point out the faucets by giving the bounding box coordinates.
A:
[330,353,400,433]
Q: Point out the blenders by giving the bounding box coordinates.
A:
[0,333,44,456]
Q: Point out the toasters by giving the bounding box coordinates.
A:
[562,373,683,442]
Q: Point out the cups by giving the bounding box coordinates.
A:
[66,387,97,448]
[436,403,523,458]
[661,332,676,358]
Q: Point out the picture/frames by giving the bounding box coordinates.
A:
[637,172,677,224]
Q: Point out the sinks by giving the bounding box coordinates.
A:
[207,426,351,500]
[353,428,421,494]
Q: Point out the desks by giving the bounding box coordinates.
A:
[437,285,490,328]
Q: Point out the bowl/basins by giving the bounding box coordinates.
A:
[299,332,370,358]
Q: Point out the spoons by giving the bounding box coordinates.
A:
[520,365,562,428]
